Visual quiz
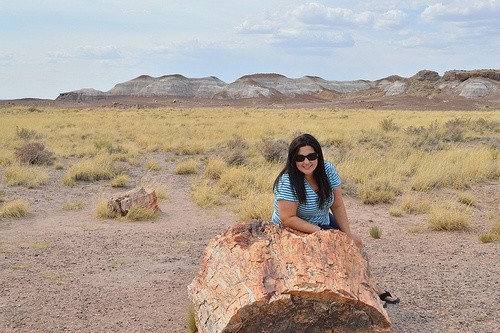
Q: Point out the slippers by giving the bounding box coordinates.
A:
[379,290,399,304]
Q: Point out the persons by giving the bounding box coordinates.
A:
[272,134,400,304]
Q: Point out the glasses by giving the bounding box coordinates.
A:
[295,152,318,162]
